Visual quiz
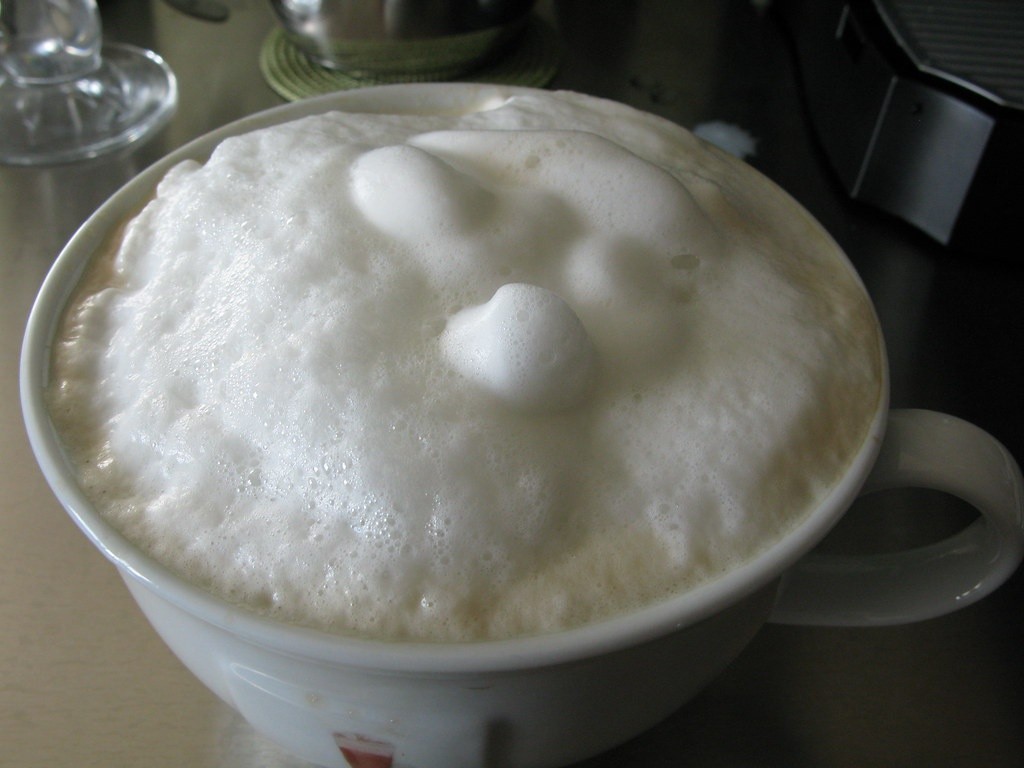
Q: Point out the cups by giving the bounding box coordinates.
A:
[263,1,537,77]
[18,81,1024,768]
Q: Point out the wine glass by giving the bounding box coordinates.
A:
[0,0,175,167]
